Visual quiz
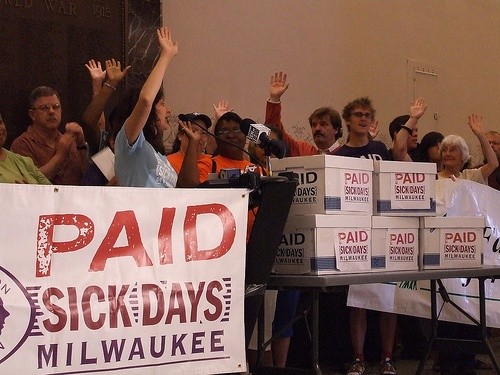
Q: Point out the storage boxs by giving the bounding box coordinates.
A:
[419,214,486,271]
[370,215,420,272]
[270,214,372,276]
[270,156,375,217]
[371,159,437,217]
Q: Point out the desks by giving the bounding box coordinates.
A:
[252,265,500,375]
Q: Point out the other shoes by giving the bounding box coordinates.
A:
[379,357,397,375]
[346,358,367,375]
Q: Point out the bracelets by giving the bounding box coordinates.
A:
[93,93,100,95]
[400,124,412,135]
[104,82,116,91]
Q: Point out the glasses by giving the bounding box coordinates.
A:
[217,126,242,134]
[32,103,63,112]
[350,112,374,119]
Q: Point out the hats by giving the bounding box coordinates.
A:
[183,115,211,129]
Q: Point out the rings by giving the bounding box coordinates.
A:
[181,127,185,130]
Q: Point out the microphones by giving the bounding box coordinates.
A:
[177,113,267,176]
[239,118,285,159]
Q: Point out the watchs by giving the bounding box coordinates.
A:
[76,142,89,151]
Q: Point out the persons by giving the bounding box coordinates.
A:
[10,86,90,185]
[0,114,52,185]
[80,27,500,375]
